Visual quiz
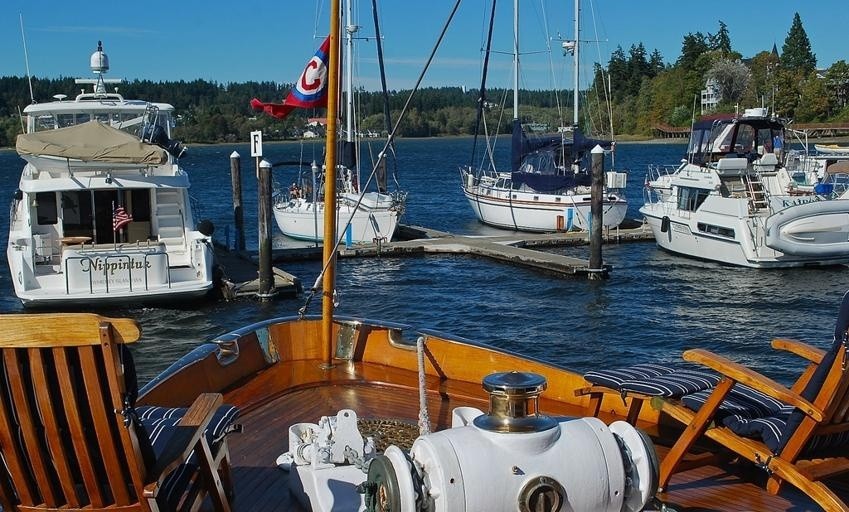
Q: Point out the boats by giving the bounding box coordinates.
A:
[129,4,828,512]
[640,94,847,270]
[7,40,214,310]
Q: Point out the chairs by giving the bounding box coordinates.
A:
[573,289,848,510]
[1,309,243,509]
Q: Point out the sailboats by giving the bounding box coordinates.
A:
[459,0,629,231]
[273,1,408,244]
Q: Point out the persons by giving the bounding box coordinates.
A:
[290,182,302,197]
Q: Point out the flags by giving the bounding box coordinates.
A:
[250,34,328,119]
[112,204,133,233]
